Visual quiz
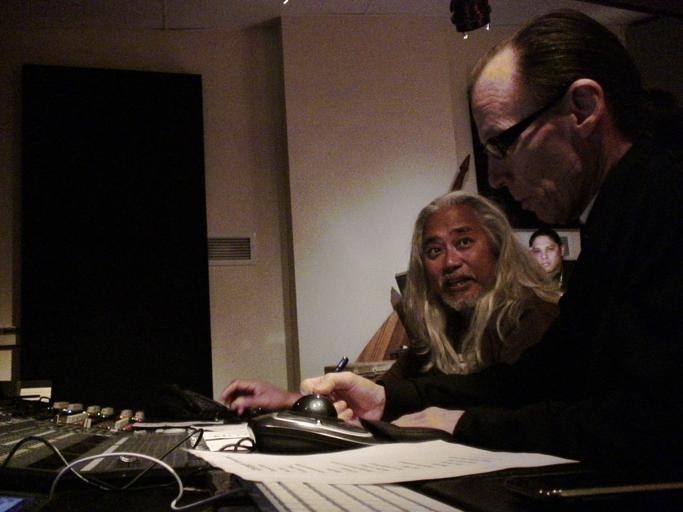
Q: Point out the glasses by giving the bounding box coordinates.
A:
[479,91,565,161]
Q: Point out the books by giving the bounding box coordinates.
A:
[322,357,397,382]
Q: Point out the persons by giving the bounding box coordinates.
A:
[298,8,683,511]
[524,229,578,294]
[221,189,561,413]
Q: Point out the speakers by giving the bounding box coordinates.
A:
[468,96,580,232]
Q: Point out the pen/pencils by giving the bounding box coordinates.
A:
[122,424,133,431]
[334,356,349,372]
